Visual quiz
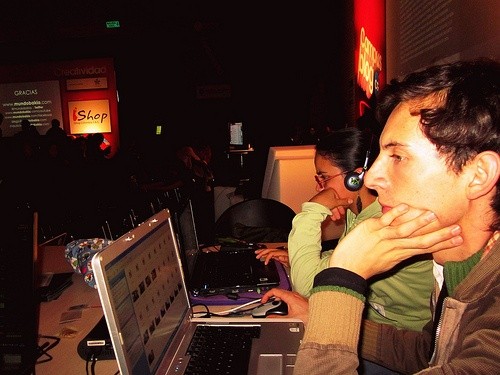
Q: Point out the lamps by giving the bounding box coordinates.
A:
[67,100,112,133]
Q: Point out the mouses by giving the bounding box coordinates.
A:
[253,299,288,318]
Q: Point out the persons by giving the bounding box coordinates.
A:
[259,52,500,375]
[0,104,254,253]
[253,114,433,337]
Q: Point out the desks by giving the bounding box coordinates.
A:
[35,242,290,375]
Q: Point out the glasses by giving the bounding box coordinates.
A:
[314,168,355,188]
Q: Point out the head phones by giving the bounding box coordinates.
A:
[345,142,371,191]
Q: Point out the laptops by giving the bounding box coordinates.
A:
[174,199,281,295]
[92,209,305,375]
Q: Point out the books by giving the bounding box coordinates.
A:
[40,270,76,304]
